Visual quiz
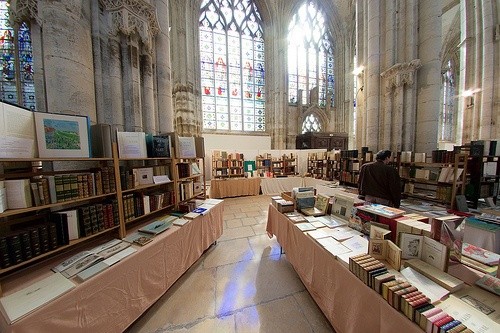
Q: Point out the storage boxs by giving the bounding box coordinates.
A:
[280,191,317,210]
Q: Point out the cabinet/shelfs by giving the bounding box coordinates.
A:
[397,151,467,210]
[209,146,400,199]
[0,143,207,297]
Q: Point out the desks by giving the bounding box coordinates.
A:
[266,187,500,333]
[398,198,499,255]
[0,196,224,332]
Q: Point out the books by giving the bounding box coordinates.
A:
[212,151,245,179]
[393,140,500,210]
[307,146,393,186]
[271,186,500,333]
[0,100,224,324]
[256,153,298,178]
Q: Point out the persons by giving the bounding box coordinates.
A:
[358,149,401,208]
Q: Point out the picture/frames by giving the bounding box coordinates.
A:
[33,111,91,158]
